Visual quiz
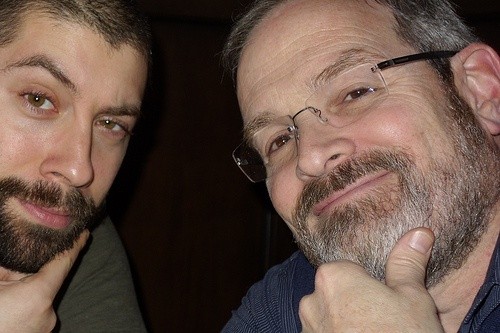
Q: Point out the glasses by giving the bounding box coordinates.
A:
[233,50,459,183]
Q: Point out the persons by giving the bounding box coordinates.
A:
[0,0,153,333]
[218,0,500,333]
